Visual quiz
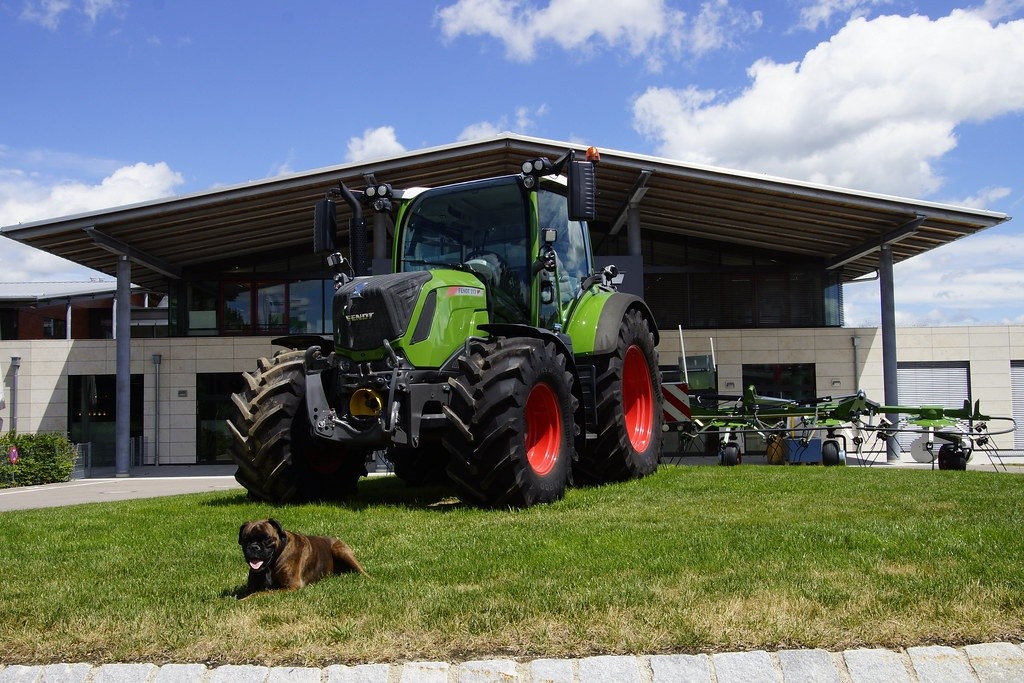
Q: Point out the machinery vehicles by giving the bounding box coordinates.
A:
[224,151,663,512]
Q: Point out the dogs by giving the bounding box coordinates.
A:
[236,518,375,600]
